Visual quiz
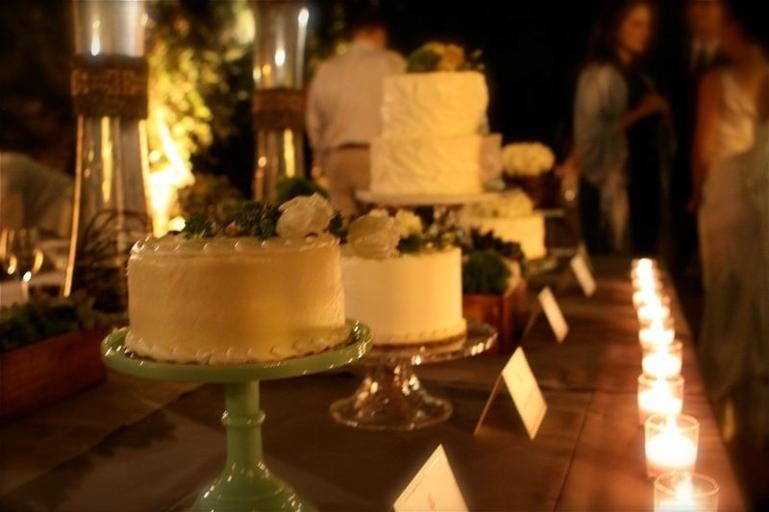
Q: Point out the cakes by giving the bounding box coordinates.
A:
[369,40,491,195]
[435,187,548,261]
[122,192,355,365]
[341,207,470,346]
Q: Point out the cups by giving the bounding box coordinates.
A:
[639,316,720,512]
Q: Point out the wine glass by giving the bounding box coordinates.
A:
[1,227,43,305]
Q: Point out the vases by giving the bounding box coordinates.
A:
[61,1,162,326]
[240,0,314,204]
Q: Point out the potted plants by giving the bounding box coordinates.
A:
[452,228,530,356]
[0,287,112,424]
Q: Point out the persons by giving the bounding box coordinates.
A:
[685,0,767,452]
[570,0,685,262]
[303,9,409,224]
[14,90,77,168]
